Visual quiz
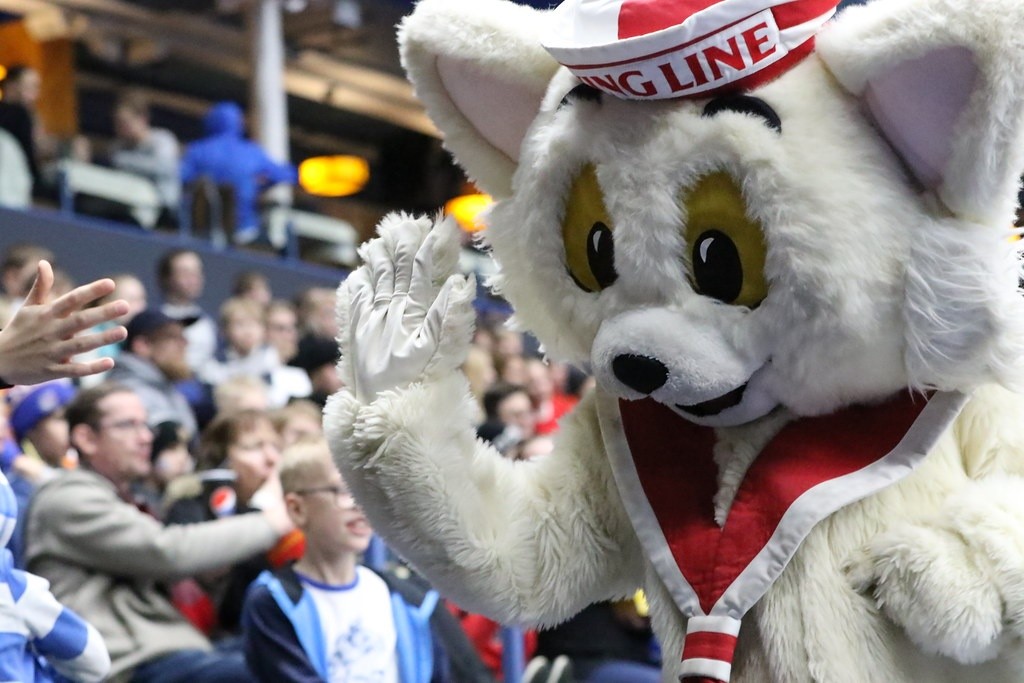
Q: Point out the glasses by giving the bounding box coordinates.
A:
[296,484,347,496]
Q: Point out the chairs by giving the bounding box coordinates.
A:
[183,174,226,250]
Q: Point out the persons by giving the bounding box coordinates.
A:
[25,386,298,683]
[1,245,661,683]
[242,441,449,683]
[179,103,297,246]
[0,65,56,202]
[111,94,180,230]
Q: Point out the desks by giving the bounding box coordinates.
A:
[260,208,356,269]
[46,158,162,232]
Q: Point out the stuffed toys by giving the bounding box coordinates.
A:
[323,1,1024,683]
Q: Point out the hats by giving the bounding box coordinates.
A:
[117,308,202,353]
[287,334,340,372]
[10,384,81,446]
